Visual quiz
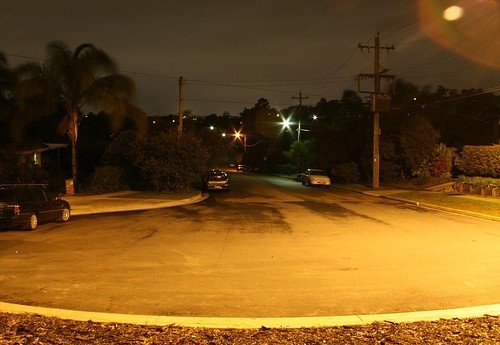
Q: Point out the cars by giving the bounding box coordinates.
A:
[238,165,244,172]
[0,184,70,230]
[201,170,231,190]
[299,169,331,187]
[229,161,235,168]
